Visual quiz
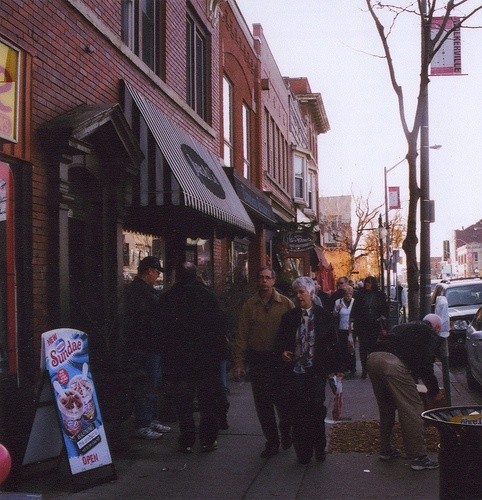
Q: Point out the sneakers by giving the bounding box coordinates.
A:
[150,423,171,433]
[380,449,400,460]
[411,457,439,470]
[134,428,163,439]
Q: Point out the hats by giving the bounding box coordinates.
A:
[143,256,165,272]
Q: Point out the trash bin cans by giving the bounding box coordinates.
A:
[420,405,482,500]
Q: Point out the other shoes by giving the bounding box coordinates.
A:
[184,447,192,453]
[315,448,326,462]
[262,448,276,458]
[281,434,292,449]
[296,456,308,463]
[202,441,218,451]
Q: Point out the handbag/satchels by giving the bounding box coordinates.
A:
[348,332,354,354]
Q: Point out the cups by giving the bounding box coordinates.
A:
[56,389,85,437]
[68,374,97,422]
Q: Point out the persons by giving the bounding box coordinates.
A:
[125,254,235,454]
[275,276,345,463]
[365,313,447,471]
[235,267,300,457]
[310,276,389,380]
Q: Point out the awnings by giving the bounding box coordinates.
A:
[121,81,257,243]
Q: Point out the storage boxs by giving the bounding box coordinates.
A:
[416,384,445,410]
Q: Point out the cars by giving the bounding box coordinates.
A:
[430,274,482,360]
[465,306,482,392]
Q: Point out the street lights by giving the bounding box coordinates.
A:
[383,142,443,303]
